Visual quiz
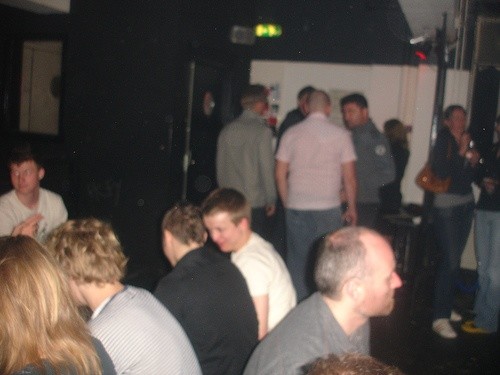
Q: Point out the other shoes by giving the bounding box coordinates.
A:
[460,324,493,333]
[433,317,457,339]
[464,321,476,325]
[450,313,462,321]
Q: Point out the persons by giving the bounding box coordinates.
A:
[277,86,314,138]
[216,84,279,238]
[243,225,402,374]
[1,151,68,242]
[41,218,203,374]
[300,351,403,374]
[340,93,396,229]
[152,203,259,374]
[460,114,500,334]
[373,118,412,242]
[199,188,297,342]
[173,88,224,206]
[274,90,358,303]
[274,85,316,258]
[430,105,481,339]
[1,234,116,374]
